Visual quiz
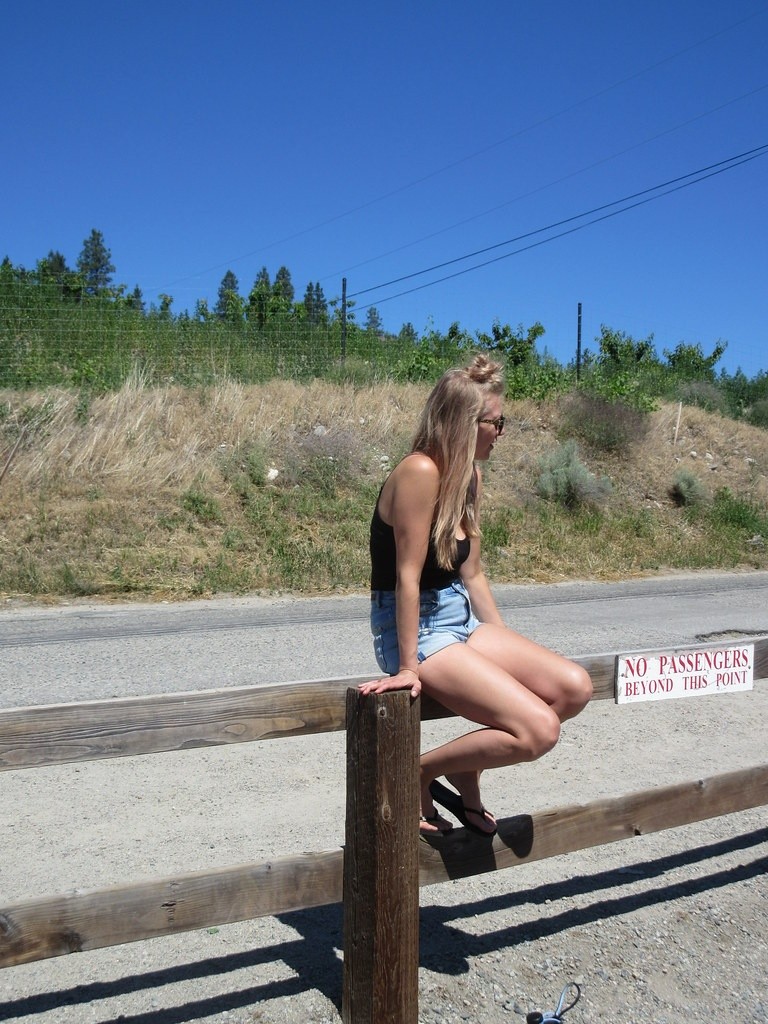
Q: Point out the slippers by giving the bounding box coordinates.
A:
[419,806,454,837]
[429,778,497,837]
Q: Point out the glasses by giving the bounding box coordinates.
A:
[477,415,505,435]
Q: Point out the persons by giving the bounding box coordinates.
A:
[357,353,594,834]
